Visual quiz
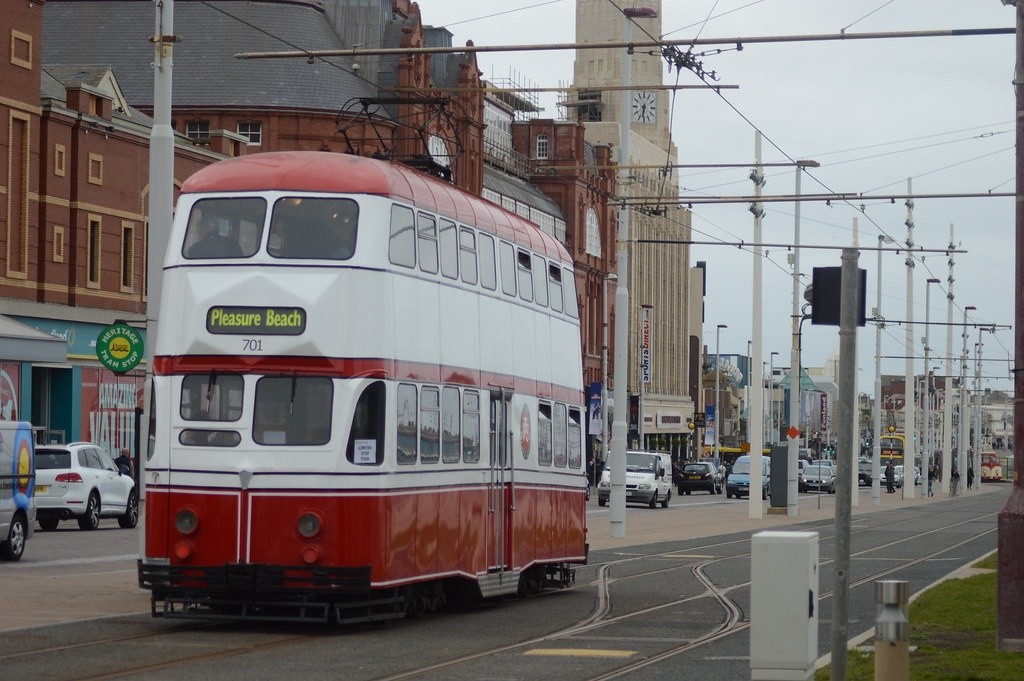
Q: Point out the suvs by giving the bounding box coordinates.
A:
[677,461,723,495]
[859,459,874,485]
[34,442,138,531]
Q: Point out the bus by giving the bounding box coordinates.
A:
[880,436,904,465]
[935,449,1002,481]
[131,152,590,624]
[704,446,771,464]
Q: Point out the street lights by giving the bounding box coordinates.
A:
[787,157,825,517]
[959,304,979,489]
[746,340,752,443]
[870,234,900,493]
[922,278,942,496]
[637,304,654,449]
[763,361,768,447]
[770,351,780,444]
[600,271,619,459]
[715,324,730,457]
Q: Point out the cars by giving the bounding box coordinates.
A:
[813,459,835,469]
[799,460,811,482]
[879,465,901,487]
[896,466,921,485]
[799,465,836,494]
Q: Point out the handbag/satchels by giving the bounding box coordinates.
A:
[971,476,974,480]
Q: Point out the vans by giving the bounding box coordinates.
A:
[726,456,771,500]
[596,449,674,508]
[0,421,37,558]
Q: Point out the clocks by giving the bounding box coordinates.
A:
[630,89,657,127]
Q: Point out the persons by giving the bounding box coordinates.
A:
[185,409,212,445]
[717,462,726,491]
[967,466,974,490]
[951,464,959,497]
[723,460,734,483]
[270,211,340,259]
[884,458,896,494]
[114,448,134,478]
[188,216,245,257]
[928,464,934,498]
[261,405,288,431]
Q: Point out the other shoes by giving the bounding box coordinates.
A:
[887,488,896,493]
[931,493,934,497]
